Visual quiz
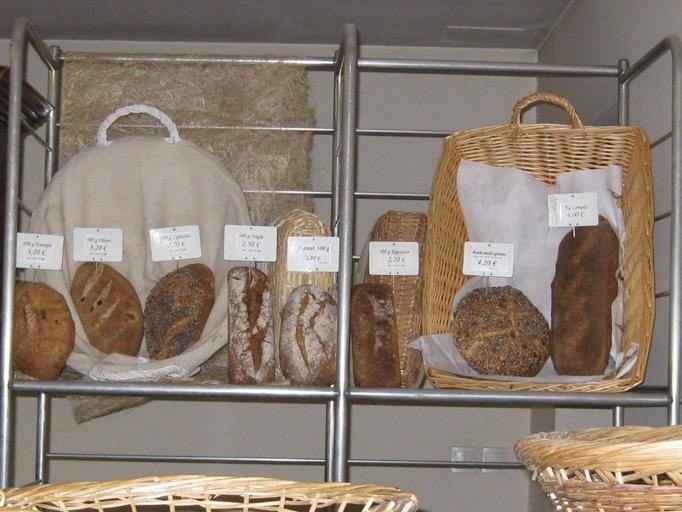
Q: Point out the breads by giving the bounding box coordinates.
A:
[143,263,215,361]
[227,266,276,386]
[449,285,551,377]
[13,279,74,379]
[349,283,402,388]
[71,261,142,357]
[551,213,620,375]
[279,284,337,387]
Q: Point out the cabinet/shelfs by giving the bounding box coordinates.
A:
[0,17,682,492]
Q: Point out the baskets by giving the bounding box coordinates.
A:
[261,206,337,387]
[354,208,430,391]
[509,422,682,512]
[0,470,422,512]
[415,89,658,399]
